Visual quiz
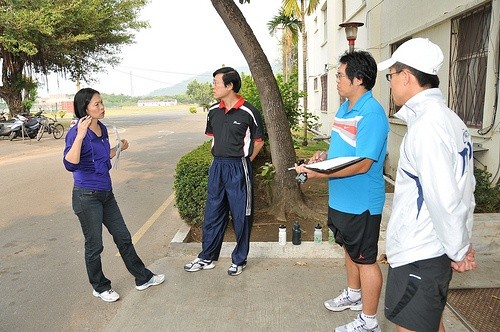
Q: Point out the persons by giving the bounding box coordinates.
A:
[377,37,478,332]
[184,66,265,276]
[295,50,391,332]
[63,88,166,302]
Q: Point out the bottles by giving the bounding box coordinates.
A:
[328,227,337,245]
[279,225,287,246]
[292,221,301,245]
[314,224,323,245]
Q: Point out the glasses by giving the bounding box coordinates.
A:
[386,70,403,81]
[336,74,350,80]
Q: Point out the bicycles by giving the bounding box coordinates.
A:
[32,116,64,141]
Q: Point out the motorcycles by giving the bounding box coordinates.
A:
[0,110,47,141]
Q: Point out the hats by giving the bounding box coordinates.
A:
[377,38,444,75]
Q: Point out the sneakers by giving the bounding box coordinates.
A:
[334,314,381,332]
[135,273,165,290]
[184,258,216,271]
[324,289,362,311]
[228,259,248,275]
[93,289,119,301]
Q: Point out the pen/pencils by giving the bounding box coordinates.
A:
[313,151,325,163]
[82,118,86,122]
